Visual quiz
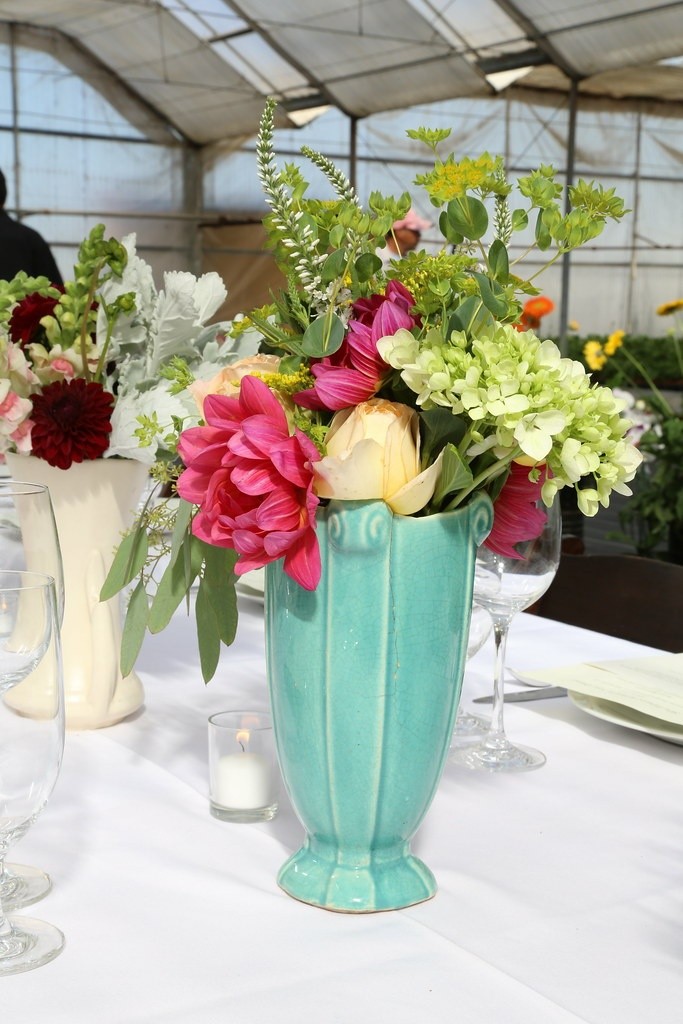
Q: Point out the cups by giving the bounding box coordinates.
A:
[207,709,278,823]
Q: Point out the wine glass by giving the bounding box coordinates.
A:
[0,477,66,910]
[0,569,66,977]
[451,605,491,737]
[470,487,562,774]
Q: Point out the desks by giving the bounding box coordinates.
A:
[3,497,682,1023]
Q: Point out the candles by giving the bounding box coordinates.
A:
[214,732,267,808]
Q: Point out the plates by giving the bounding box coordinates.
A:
[235,585,264,604]
[567,690,683,747]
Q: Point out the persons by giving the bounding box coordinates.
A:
[0,173,65,297]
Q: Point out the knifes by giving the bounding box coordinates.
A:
[472,687,569,703]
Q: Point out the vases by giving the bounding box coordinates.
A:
[262,491,496,910]
[5,447,145,724]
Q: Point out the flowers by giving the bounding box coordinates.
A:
[0,224,281,469]
[97,101,645,686]
